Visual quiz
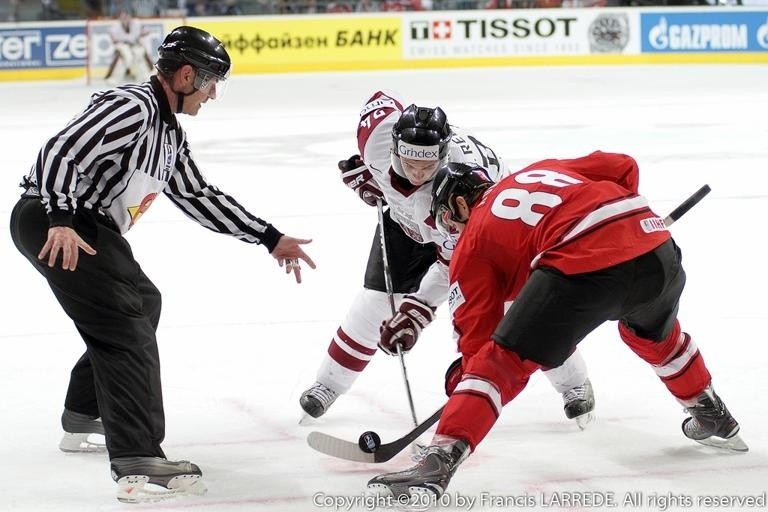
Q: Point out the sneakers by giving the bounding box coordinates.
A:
[298,383,336,420]
[110,455,204,484]
[561,379,597,419]
[60,409,107,437]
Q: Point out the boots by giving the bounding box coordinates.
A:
[368,431,470,503]
[682,395,739,442]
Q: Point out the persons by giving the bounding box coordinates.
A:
[367,149,741,505]
[299,91,595,418]
[10,26,317,492]
[1,0,743,24]
[104,10,155,79]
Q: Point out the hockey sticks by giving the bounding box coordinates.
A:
[307,184,710,463]
[377,198,426,454]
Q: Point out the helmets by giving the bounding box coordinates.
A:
[429,160,495,247]
[156,26,232,101]
[391,104,454,162]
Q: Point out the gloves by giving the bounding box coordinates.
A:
[378,293,438,359]
[341,154,390,208]
